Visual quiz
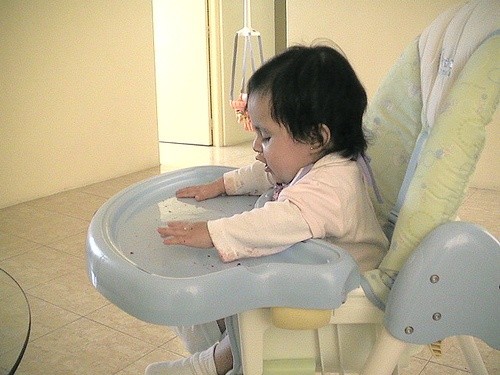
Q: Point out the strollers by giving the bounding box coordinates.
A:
[86,0,500,375]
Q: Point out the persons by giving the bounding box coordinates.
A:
[145,35,390,375]
